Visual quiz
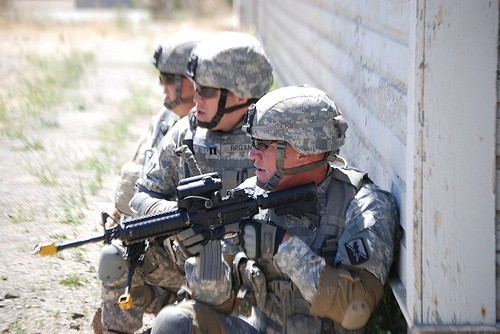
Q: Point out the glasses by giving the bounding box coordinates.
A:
[158,75,184,85]
[194,82,218,99]
[249,137,273,151]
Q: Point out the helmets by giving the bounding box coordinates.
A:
[241,84,348,155]
[186,31,274,99]
[151,31,205,76]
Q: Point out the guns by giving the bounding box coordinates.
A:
[32,173,320,313]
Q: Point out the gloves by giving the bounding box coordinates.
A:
[238,216,287,261]
[175,224,211,258]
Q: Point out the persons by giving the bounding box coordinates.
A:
[97,31,400,334]
